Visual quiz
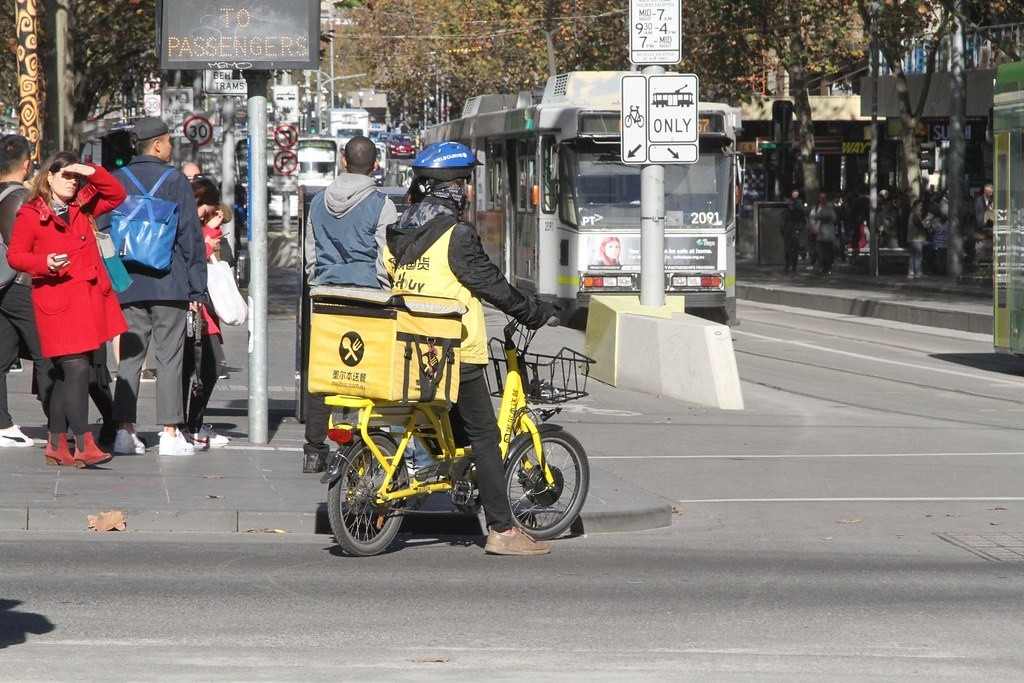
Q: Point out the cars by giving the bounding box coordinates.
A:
[268,176,300,219]
[366,130,418,188]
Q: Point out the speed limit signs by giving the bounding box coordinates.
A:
[143,95,162,116]
[183,116,212,146]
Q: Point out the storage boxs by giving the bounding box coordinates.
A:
[305,283,469,403]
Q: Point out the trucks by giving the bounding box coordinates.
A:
[327,108,369,154]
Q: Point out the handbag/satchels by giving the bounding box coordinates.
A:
[207,253,248,326]
[75,196,134,293]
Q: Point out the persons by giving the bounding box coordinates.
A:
[6,152,126,468]
[879,184,994,278]
[0,134,78,451]
[599,237,622,267]
[301,136,398,476]
[93,116,206,455]
[178,159,236,445]
[780,189,870,276]
[381,140,556,555]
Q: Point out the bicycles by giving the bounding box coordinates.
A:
[319,300,596,557]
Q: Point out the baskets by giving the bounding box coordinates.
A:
[482,337,597,403]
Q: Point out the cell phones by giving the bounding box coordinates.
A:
[54,254,67,263]
[216,232,230,239]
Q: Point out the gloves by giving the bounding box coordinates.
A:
[531,302,559,330]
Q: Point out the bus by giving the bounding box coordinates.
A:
[266,137,338,188]
[421,29,740,327]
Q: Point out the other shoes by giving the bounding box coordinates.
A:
[219,370,230,378]
[303,443,330,473]
[9,359,23,372]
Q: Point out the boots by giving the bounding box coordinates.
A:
[45,431,76,467]
[74,431,112,468]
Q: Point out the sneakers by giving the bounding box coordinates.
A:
[181,424,230,446]
[158,428,195,456]
[140,369,157,383]
[113,429,145,454]
[484,526,552,555]
[0,424,34,447]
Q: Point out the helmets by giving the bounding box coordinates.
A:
[411,141,484,181]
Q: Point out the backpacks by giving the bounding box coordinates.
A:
[110,167,180,272]
[0,184,28,289]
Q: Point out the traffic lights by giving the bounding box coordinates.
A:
[109,130,132,171]
[308,117,320,136]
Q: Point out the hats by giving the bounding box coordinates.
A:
[130,117,168,143]
